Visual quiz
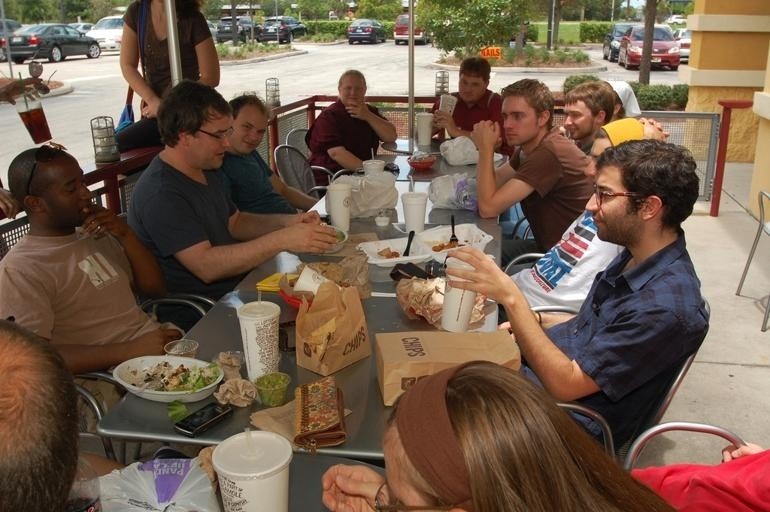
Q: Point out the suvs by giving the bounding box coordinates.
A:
[0,20,23,63]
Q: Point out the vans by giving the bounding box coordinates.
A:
[393,15,427,44]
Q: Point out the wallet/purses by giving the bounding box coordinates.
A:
[294,376,346,447]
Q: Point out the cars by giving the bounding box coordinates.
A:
[9,24,101,64]
[71,23,91,33]
[262,17,306,43]
[86,16,125,51]
[619,26,679,71]
[602,24,630,62]
[675,29,692,63]
[206,19,217,36]
[667,14,687,25]
[217,16,261,42]
[346,18,387,44]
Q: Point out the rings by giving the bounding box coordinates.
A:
[97,225,101,229]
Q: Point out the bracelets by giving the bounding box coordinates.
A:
[534,310,543,323]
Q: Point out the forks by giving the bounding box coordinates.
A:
[449,214,458,246]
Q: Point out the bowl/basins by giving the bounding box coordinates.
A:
[407,155,436,170]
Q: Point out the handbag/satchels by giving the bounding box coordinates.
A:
[119,103,135,124]
[374,330,521,406]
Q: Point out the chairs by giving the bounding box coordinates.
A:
[273,144,332,197]
[116,211,221,306]
[68,295,206,472]
[287,127,355,192]
[735,189,770,334]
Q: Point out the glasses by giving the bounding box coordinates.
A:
[24,142,67,192]
[29,48,57,95]
[197,127,234,141]
[374,483,451,511]
[592,182,647,206]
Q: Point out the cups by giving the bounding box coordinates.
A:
[438,93,458,117]
[438,258,478,333]
[253,371,291,409]
[415,112,434,146]
[18,100,52,144]
[235,300,282,383]
[327,183,353,232]
[209,429,295,512]
[162,339,199,358]
[401,192,428,233]
[362,160,385,176]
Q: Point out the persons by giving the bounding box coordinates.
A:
[0,180,19,220]
[123,79,340,329]
[562,84,616,157]
[497,117,672,337]
[221,95,321,225]
[0,320,82,512]
[442,138,710,454]
[113,0,221,155]
[0,146,192,434]
[303,69,398,192]
[591,79,642,123]
[428,56,516,162]
[629,440,770,511]
[320,359,679,511]
[470,79,597,276]
[0,76,42,106]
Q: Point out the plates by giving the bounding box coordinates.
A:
[356,236,433,268]
[113,354,225,404]
[418,222,495,264]
[279,280,312,309]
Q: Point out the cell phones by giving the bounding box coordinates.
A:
[173,402,232,437]
